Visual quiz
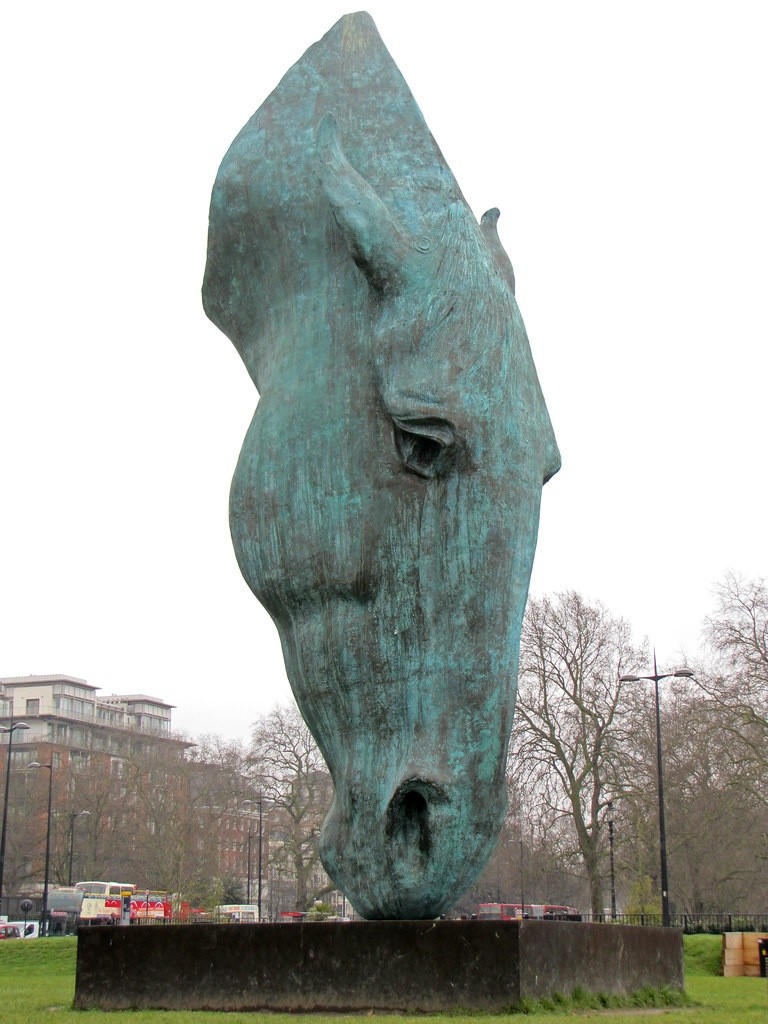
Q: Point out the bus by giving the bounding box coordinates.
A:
[478,902,578,923]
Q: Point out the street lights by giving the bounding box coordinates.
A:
[28,743,54,927]
[53,804,91,884]
[243,793,263,929]
[0,710,31,896]
[618,646,698,930]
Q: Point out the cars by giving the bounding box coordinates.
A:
[0,878,298,940]
